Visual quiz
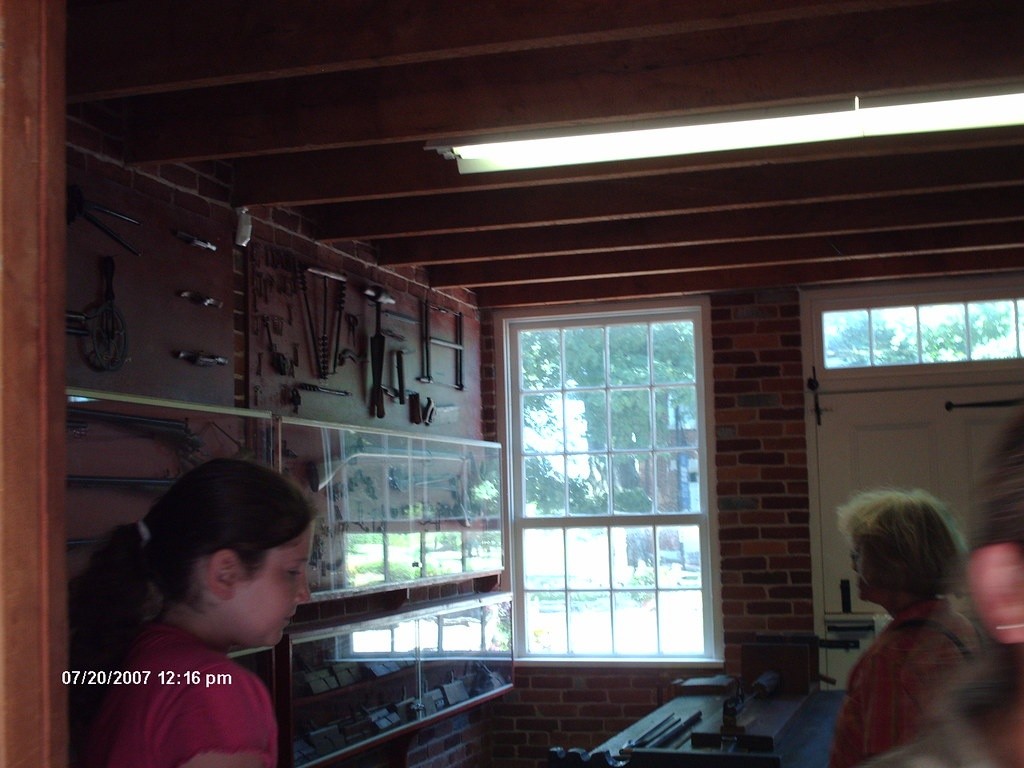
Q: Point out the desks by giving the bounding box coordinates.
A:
[584,675,847,768]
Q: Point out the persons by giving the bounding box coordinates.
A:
[827,407,1024,768]
[66,458,315,768]
